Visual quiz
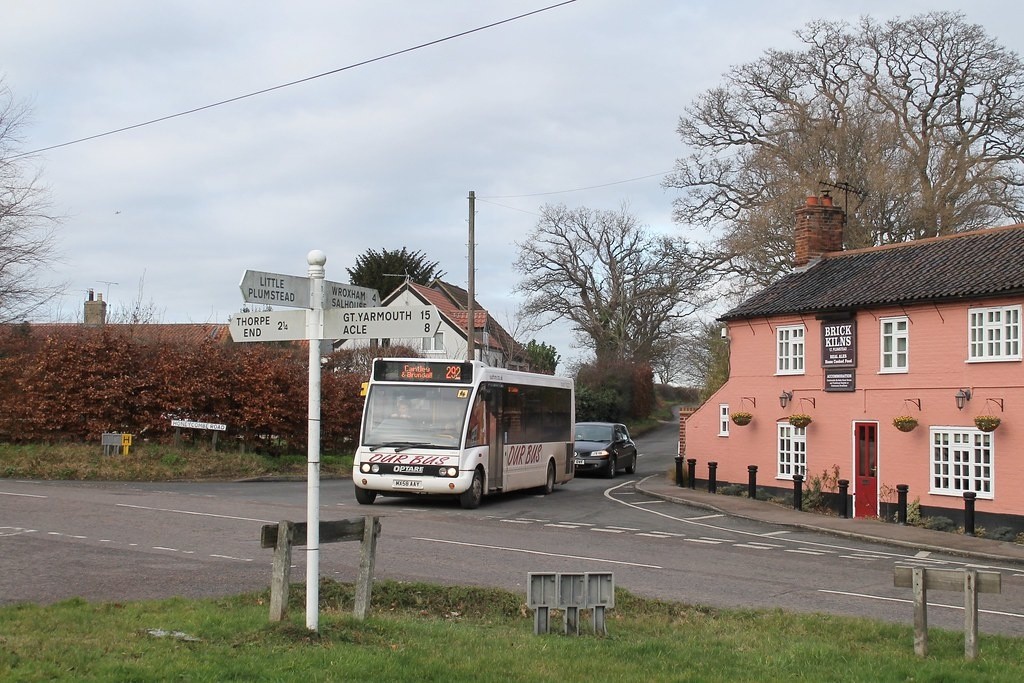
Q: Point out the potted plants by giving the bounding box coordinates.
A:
[973,415,1002,432]
[730,412,753,425]
[892,415,919,432]
[788,414,812,428]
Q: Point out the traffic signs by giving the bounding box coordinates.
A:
[228,305,441,344]
[239,269,381,308]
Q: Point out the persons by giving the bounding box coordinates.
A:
[392,400,409,417]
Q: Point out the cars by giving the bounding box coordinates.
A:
[574,422,637,478]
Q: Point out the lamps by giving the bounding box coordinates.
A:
[779,390,792,409]
[955,388,970,412]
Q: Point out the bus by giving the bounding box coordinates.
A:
[351,356,576,510]
